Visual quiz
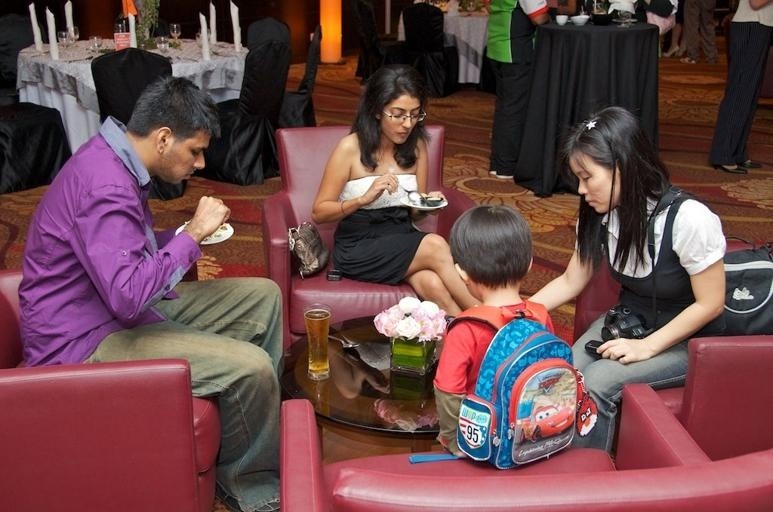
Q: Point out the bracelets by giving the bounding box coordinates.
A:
[337,199,349,219]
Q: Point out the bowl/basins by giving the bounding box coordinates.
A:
[424,197,443,206]
[570,15,590,26]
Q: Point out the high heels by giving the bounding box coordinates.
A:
[663,46,679,58]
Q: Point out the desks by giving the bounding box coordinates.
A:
[515,18,659,200]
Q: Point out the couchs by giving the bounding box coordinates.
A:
[260,125,479,351]
[278,383,773,511]
[0,269,222,511]
[569,239,772,461]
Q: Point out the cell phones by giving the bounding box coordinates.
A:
[584,339,603,353]
[326,269,342,281]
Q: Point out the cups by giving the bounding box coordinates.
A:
[302,307,334,380]
[555,15,568,26]
[114,20,126,43]
[155,37,168,54]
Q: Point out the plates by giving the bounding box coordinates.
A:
[173,221,235,246]
[403,193,449,214]
[611,18,639,24]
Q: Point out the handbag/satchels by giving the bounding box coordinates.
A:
[704,235,773,336]
[289,221,328,279]
[648,0,674,18]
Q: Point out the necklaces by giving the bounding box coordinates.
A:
[378,155,400,173]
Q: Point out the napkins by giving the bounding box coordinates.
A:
[207,3,217,39]
[64,1,75,44]
[46,7,58,50]
[28,4,43,52]
[197,12,207,35]
[127,12,138,47]
[228,2,244,47]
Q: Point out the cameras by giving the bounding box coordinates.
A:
[601,305,652,342]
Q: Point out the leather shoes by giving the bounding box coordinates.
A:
[713,164,747,174]
[741,160,761,168]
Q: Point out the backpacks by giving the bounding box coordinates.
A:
[449,301,599,477]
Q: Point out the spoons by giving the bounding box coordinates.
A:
[344,347,360,362]
[389,174,424,205]
[327,334,361,349]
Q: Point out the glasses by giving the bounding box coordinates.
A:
[382,109,427,124]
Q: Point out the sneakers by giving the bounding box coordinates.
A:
[680,57,696,65]
[214,480,280,511]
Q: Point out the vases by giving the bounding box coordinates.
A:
[388,334,439,374]
[389,370,435,407]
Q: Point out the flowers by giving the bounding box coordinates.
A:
[372,295,451,339]
[373,396,439,435]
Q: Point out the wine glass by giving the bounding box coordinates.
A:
[194,27,212,57]
[168,23,182,46]
[57,27,79,55]
[86,35,103,59]
[615,10,631,28]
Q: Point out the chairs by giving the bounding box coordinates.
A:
[219,20,289,117]
[279,29,326,125]
[2,12,48,100]
[90,49,190,198]
[191,40,291,185]
[402,4,460,97]
[349,2,403,85]
[0,101,74,196]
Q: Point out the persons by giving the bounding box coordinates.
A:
[309,61,482,316]
[526,104,727,457]
[432,201,557,465]
[19,75,288,512]
[453,0,773,183]
